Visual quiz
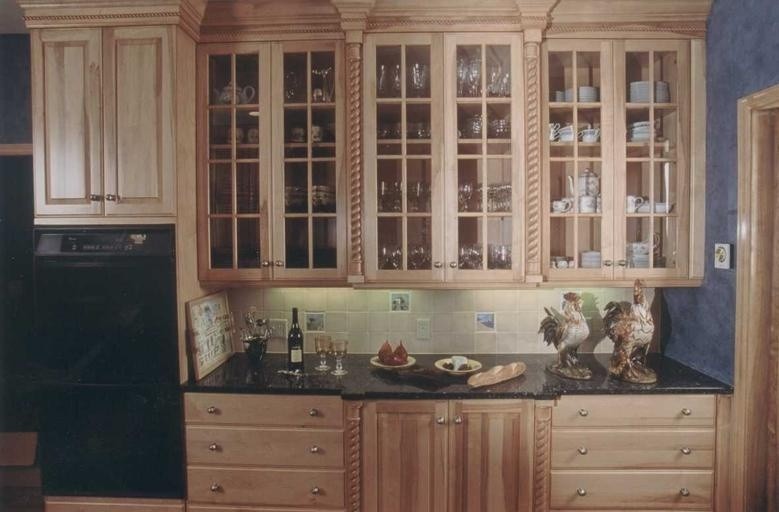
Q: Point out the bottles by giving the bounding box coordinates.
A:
[286,307,304,368]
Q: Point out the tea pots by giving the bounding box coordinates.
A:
[568,168,602,197]
[212,79,256,106]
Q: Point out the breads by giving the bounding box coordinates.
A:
[467,362,526,388]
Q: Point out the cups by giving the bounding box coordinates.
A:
[549,120,600,144]
[286,184,334,214]
[228,125,325,146]
[551,194,673,216]
[375,62,512,271]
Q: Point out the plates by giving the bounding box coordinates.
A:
[552,237,665,270]
[370,354,416,369]
[550,80,671,103]
[468,361,527,388]
[433,357,483,376]
[216,176,257,215]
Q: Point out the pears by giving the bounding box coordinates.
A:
[378,342,408,366]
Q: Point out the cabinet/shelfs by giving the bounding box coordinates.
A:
[350,0,534,291]
[197,0,349,291]
[549,391,731,511]
[183,386,350,512]
[340,391,551,511]
[16,0,207,227]
[533,0,712,291]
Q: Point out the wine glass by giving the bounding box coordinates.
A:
[331,339,348,376]
[314,334,332,371]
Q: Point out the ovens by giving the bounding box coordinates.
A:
[31,222,188,500]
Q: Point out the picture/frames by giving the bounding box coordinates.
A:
[184,290,237,383]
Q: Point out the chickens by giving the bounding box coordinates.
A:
[602,280,655,378]
[538,292,590,369]
